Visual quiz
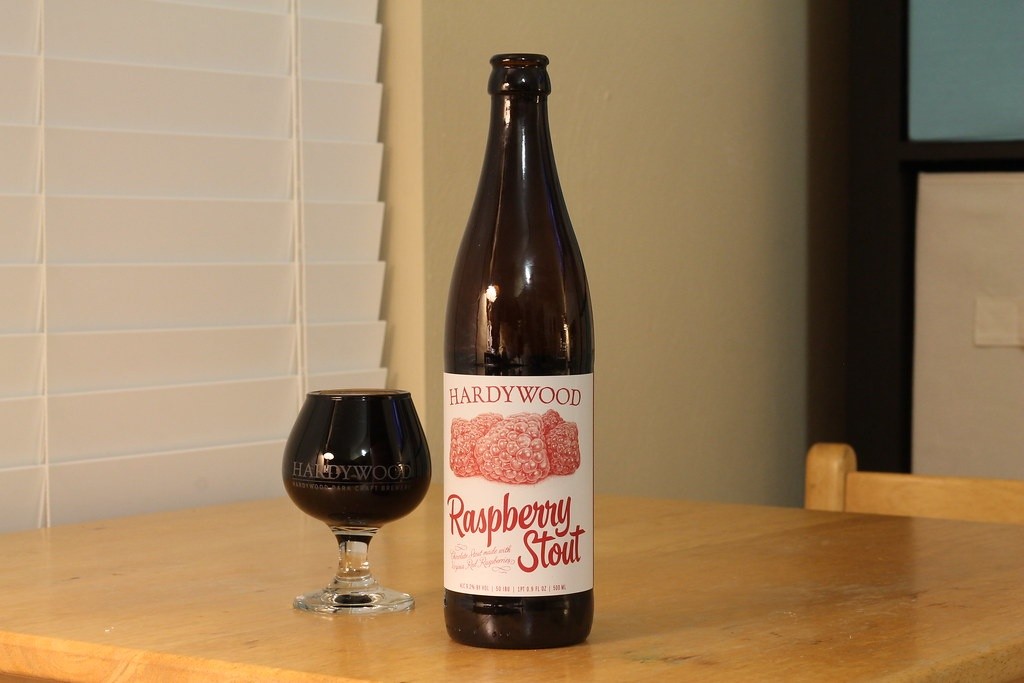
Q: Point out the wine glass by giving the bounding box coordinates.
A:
[281,390,433,616]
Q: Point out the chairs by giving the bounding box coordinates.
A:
[805,442,1024,525]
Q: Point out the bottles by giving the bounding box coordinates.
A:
[444,52,595,648]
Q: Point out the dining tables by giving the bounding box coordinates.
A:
[0,484,1024,683]
